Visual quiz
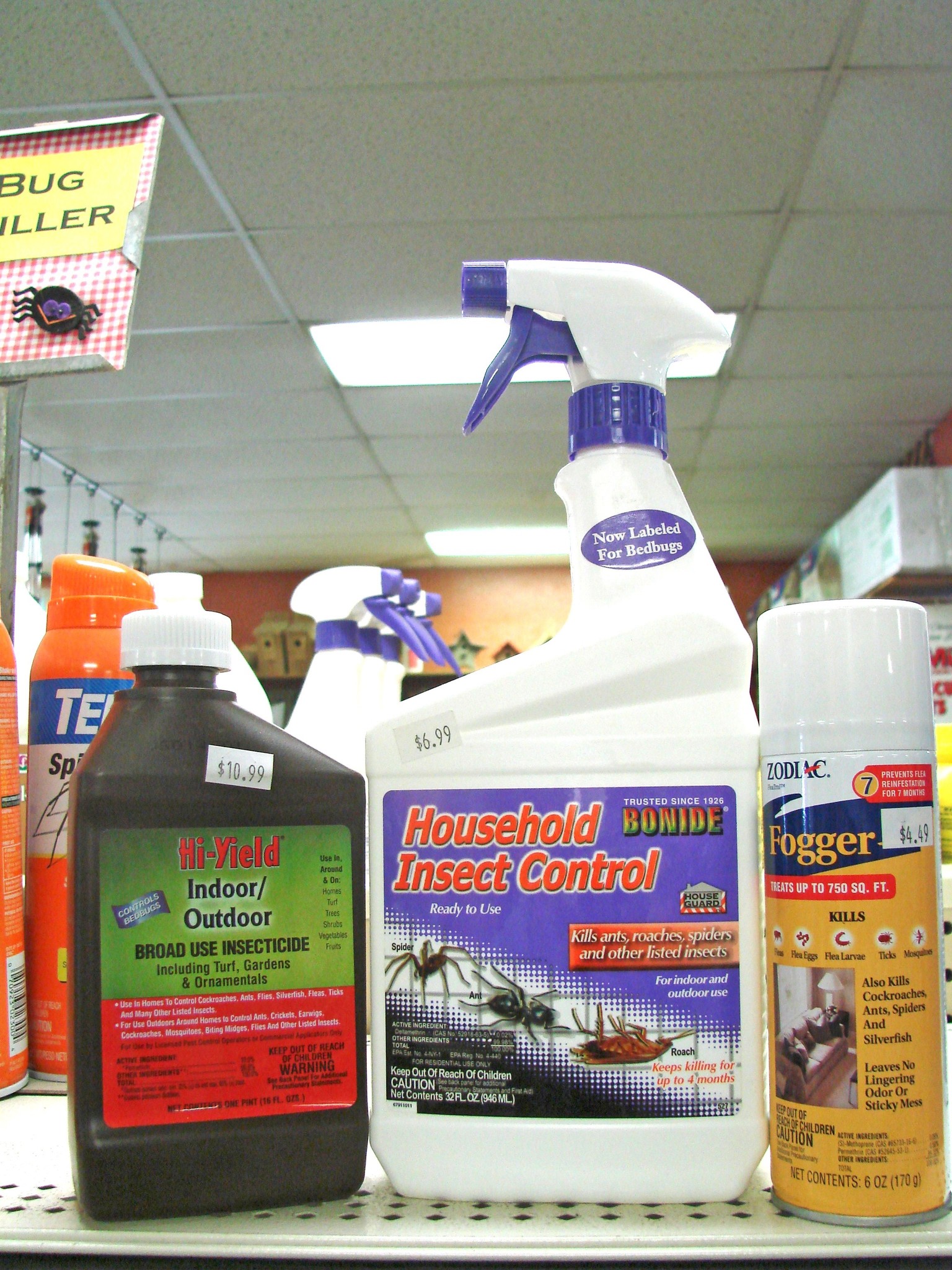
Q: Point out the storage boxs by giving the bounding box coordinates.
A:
[253,611,314,676]
